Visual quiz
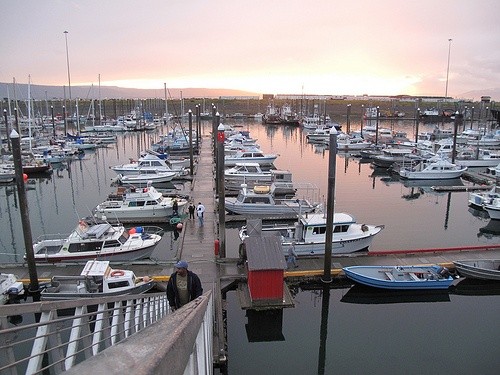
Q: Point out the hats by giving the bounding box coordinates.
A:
[174,260,189,269]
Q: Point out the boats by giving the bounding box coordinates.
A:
[453,257,500,282]
[23,210,163,267]
[217,164,298,198]
[0,113,199,183]
[238,213,386,258]
[90,181,187,223]
[41,259,157,310]
[260,100,302,125]
[342,262,456,293]
[0,274,25,308]
[215,183,314,218]
[302,106,500,224]
[224,110,278,165]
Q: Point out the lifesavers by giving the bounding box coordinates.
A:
[111,271,124,277]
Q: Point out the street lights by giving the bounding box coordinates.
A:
[2,101,68,144]
[189,103,201,180]
[321,126,338,284]
[344,99,496,150]
[9,129,40,297]
[445,39,452,102]
[212,102,228,258]
[64,31,74,136]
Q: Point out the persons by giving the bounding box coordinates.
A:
[166,260,203,313]
[188,203,195,221]
[196,202,205,227]
[287,242,299,268]
[173,200,178,213]
